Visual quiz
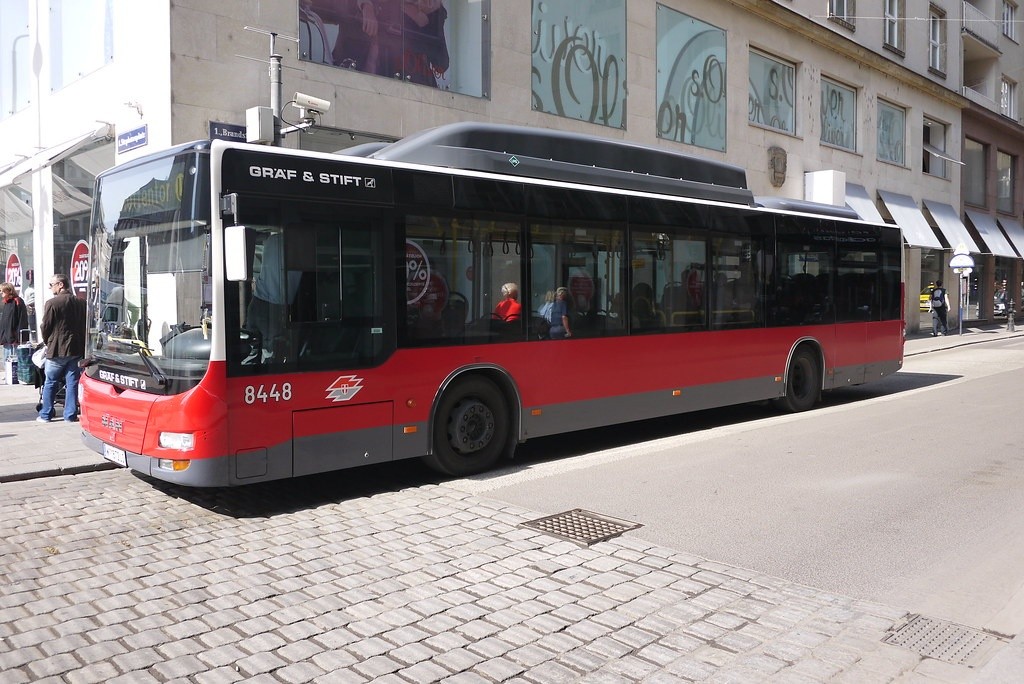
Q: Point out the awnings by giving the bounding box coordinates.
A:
[845,182,1024,260]
[0,139,116,241]
[0,119,114,188]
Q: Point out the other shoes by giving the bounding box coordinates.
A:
[75,416,79,421]
[36,416,51,423]
[934,333,937,337]
[942,331,948,336]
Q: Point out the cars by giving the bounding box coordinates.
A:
[919,284,935,311]
[976,289,1024,317]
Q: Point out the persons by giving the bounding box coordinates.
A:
[537,287,574,340]
[0,270,41,383]
[299,0,449,88]
[928,280,951,337]
[240,224,304,365]
[36,274,87,423]
[492,283,522,323]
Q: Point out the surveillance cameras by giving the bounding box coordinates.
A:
[292,92,330,115]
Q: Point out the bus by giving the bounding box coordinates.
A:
[77,112,908,491]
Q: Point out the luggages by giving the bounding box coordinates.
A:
[17,329,37,384]
[5,344,18,385]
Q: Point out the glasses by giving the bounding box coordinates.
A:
[50,282,60,287]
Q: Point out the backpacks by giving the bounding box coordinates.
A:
[932,288,944,307]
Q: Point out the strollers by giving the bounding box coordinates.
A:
[30,341,81,414]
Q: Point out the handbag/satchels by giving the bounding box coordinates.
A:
[31,342,48,369]
[537,319,552,341]
